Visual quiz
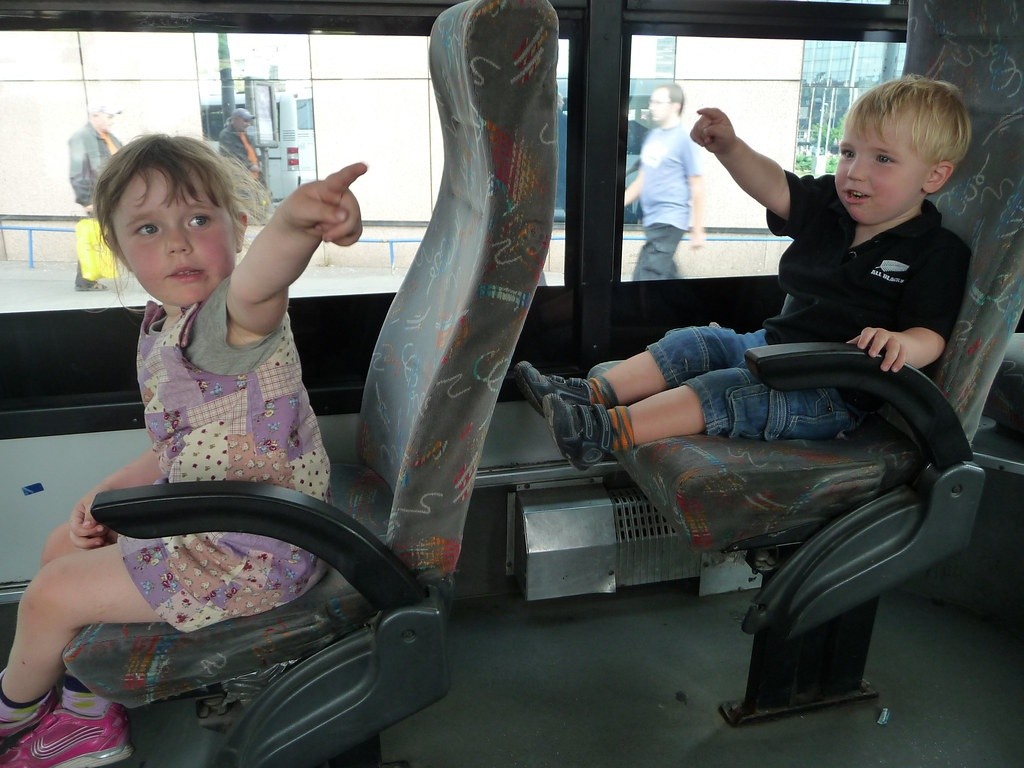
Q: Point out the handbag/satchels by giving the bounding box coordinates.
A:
[74,217,121,281]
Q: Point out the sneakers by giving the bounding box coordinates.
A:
[0,703,135,768]
[0,668,58,747]
[513,361,592,421]
[542,393,613,472]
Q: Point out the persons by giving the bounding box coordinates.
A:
[220,109,260,186]
[624,82,704,280]
[513,77,972,474]
[68,104,124,292]
[0,133,367,768]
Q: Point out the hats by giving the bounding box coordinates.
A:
[231,109,256,121]
[88,99,122,115]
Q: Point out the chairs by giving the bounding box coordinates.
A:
[595,0,1024,730]
[59,1,562,768]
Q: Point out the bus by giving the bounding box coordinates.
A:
[201,76,309,199]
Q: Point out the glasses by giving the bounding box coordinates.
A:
[649,100,672,108]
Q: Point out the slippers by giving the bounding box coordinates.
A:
[75,283,108,291]
